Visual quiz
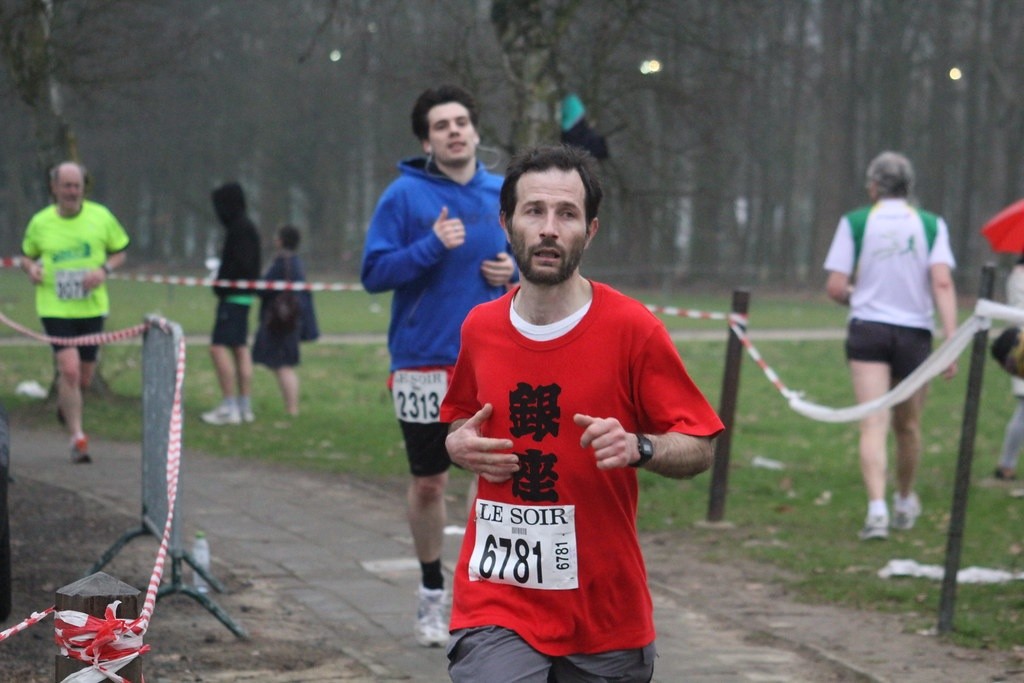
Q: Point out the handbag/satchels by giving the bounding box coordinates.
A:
[992,326,1024,379]
[266,294,296,339]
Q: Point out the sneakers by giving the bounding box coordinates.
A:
[198,406,241,426]
[413,580,449,648]
[243,407,256,424]
[70,438,92,464]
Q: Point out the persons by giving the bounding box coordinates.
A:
[20,161,130,465]
[200,181,319,427]
[440,145,724,683]
[991,247,1024,499]
[821,152,960,543]
[360,85,519,647]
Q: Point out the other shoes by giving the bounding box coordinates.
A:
[994,466,1017,482]
[856,513,890,543]
[891,491,922,530]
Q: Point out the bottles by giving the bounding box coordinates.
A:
[194,531,210,593]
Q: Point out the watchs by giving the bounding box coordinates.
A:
[628,434,653,468]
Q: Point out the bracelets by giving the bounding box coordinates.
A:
[102,264,112,274]
